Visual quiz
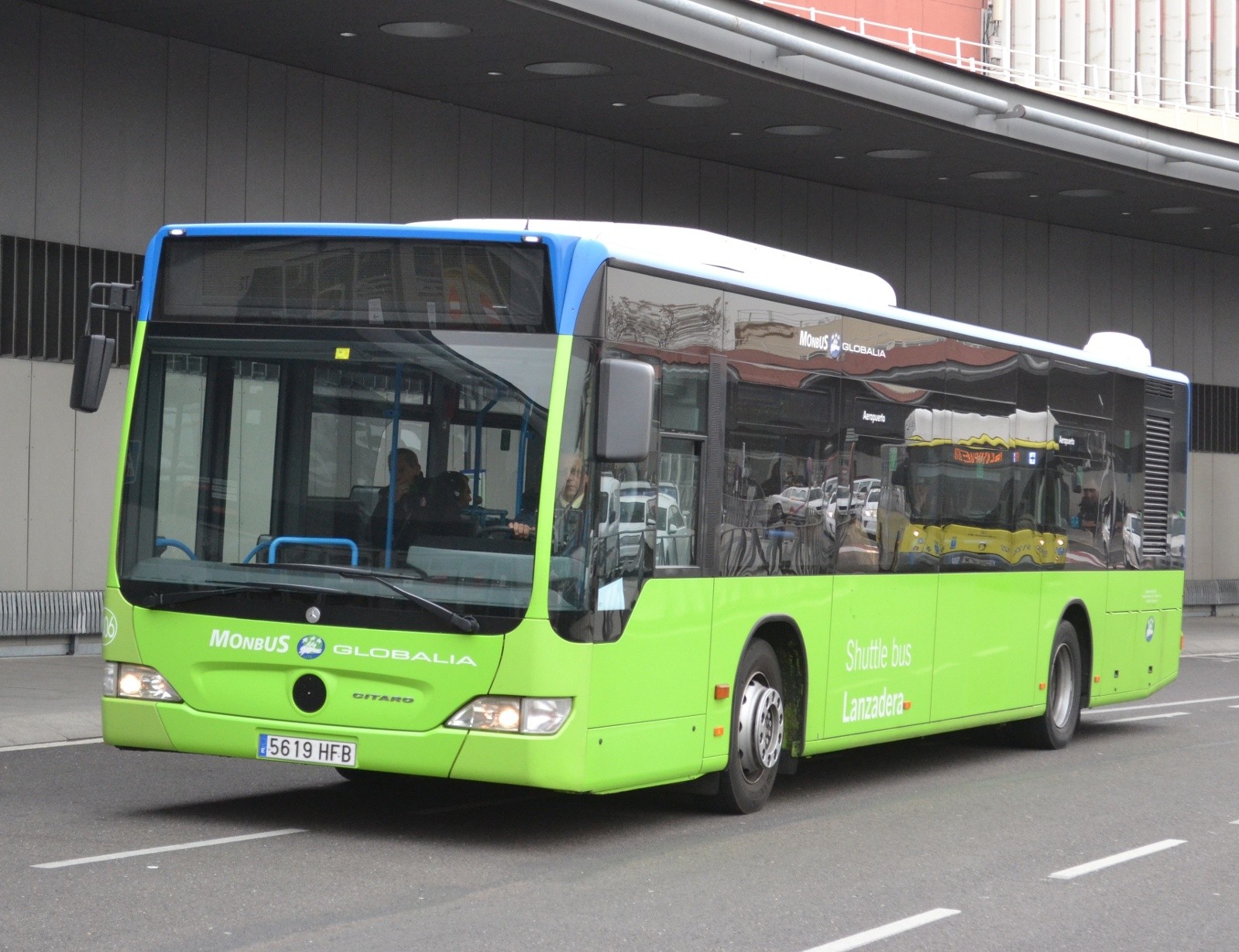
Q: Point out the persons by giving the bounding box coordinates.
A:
[509,447,588,562]
[363,448,493,569]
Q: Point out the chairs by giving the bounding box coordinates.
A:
[349,486,381,527]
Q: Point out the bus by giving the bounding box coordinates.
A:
[69,219,1194,816]
